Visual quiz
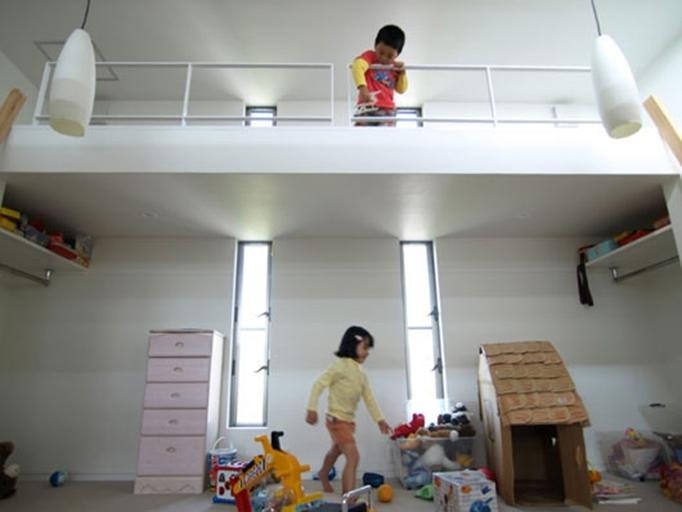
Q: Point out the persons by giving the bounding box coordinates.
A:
[353,24,408,128]
[306,326,393,506]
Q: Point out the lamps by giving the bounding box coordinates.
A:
[50,0,96,137]
[589,0,645,139]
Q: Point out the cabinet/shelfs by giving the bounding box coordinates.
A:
[134,330,227,498]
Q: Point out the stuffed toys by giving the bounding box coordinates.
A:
[388,401,479,491]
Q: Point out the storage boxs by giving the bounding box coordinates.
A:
[432,470,498,512]
[617,438,661,466]
[392,437,476,490]
